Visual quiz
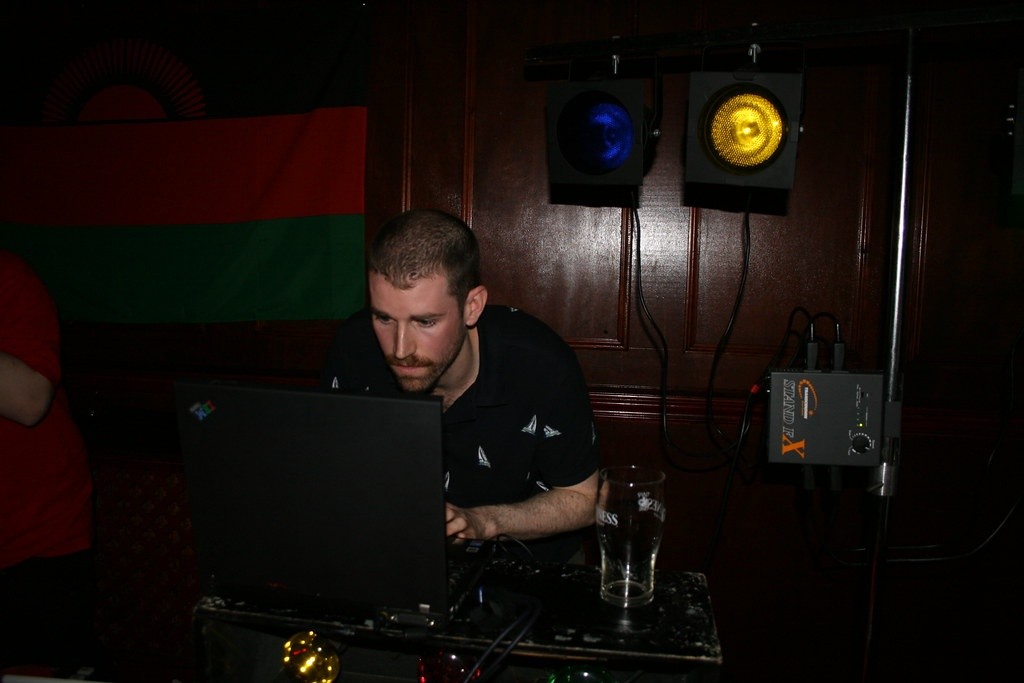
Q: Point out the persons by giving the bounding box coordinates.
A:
[0,249,95,667]
[323,206,600,590]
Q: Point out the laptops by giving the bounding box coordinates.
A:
[176,383,497,629]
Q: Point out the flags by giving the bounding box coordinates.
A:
[0,0,376,325]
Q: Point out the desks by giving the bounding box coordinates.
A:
[193,542,722,683]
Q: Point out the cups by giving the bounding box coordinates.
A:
[596,465,667,608]
